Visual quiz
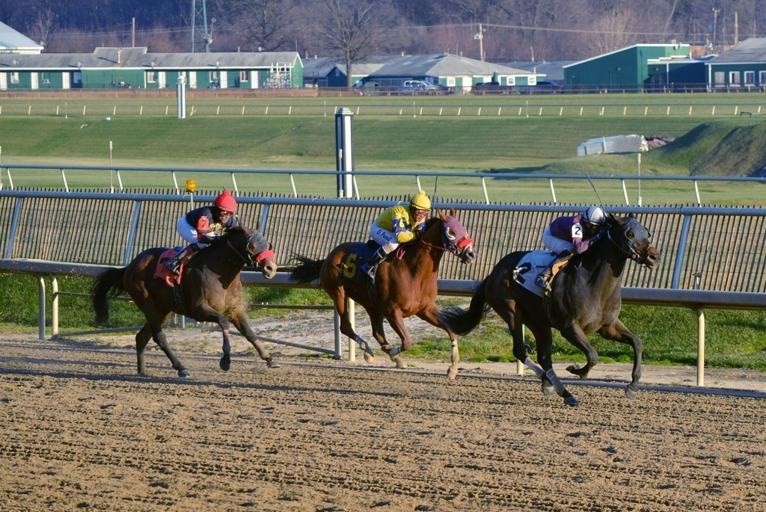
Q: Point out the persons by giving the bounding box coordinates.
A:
[535,206,607,291]
[164,190,237,275]
[361,191,431,279]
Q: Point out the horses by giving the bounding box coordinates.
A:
[286,206,477,372]
[83,221,280,377]
[435,212,659,406]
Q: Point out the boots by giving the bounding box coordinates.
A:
[165,243,199,276]
[362,246,387,280]
[535,249,573,292]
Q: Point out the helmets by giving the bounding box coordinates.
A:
[214,191,238,213]
[410,191,431,210]
[583,207,603,226]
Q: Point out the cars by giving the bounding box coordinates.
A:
[537,80,560,90]
[403,80,440,96]
[473,81,500,91]
